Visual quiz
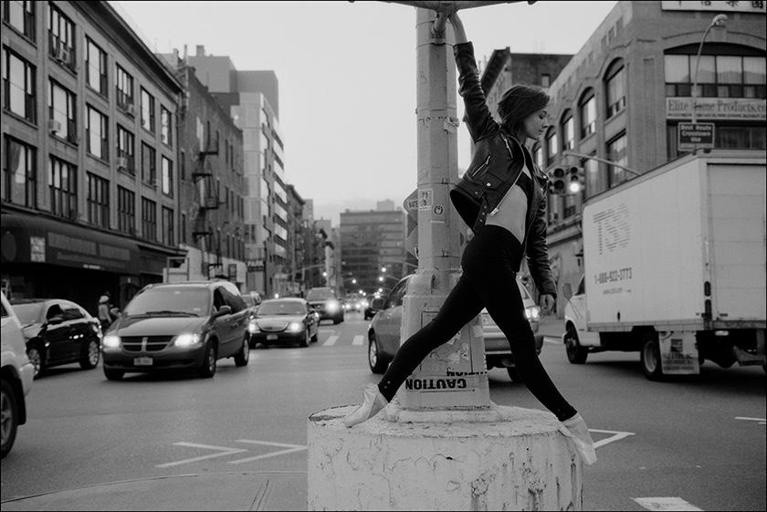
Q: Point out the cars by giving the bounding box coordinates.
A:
[365,270,540,373]
[242,290,261,306]
[0,287,35,459]
[9,297,102,377]
[306,287,345,324]
[246,297,320,347]
[102,282,250,380]
[337,285,390,321]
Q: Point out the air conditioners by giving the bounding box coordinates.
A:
[57,49,69,63]
[49,120,60,131]
[125,104,137,118]
[118,158,128,168]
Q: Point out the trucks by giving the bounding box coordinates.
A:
[563,147,767,381]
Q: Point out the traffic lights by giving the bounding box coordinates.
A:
[555,168,566,194]
[568,167,584,193]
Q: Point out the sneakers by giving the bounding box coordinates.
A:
[343,385,387,427]
[558,416,596,465]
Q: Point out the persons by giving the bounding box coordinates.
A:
[344,10,599,472]
[97,294,114,334]
[108,304,120,321]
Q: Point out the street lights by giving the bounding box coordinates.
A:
[690,14,727,121]
[559,151,640,175]
